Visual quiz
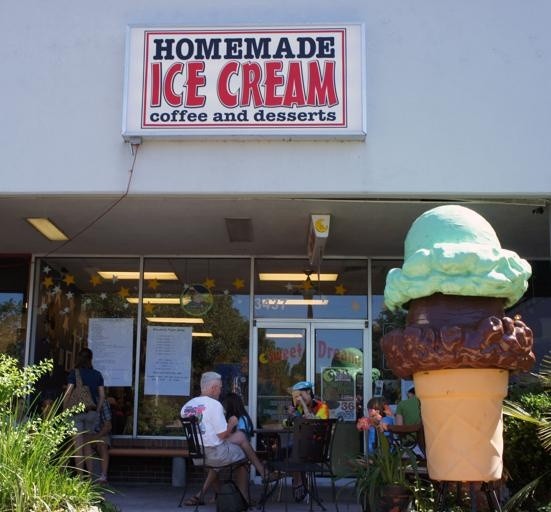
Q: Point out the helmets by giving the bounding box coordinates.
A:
[293,381,312,390]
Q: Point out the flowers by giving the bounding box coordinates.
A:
[342,405,411,512]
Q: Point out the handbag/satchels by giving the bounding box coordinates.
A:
[217,482,247,511]
[62,387,95,416]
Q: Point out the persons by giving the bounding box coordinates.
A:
[283,382,329,501]
[180,371,283,507]
[184,392,255,506]
[7,348,113,487]
[367,386,421,462]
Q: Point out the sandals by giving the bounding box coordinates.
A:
[184,496,205,506]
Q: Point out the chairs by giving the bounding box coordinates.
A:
[256,417,340,512]
[178,416,253,511]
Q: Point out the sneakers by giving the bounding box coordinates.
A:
[263,471,283,482]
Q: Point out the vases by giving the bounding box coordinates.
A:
[376,484,409,511]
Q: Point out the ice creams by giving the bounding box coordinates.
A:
[379,204,537,483]
[321,347,381,394]
